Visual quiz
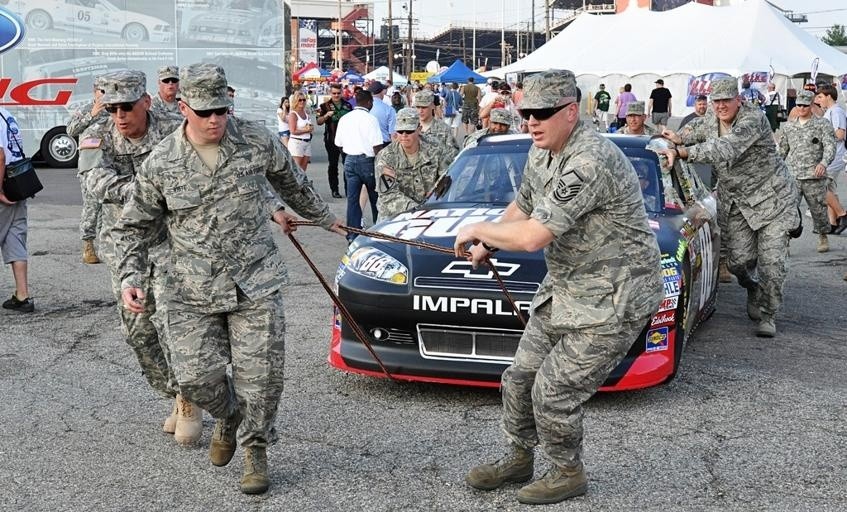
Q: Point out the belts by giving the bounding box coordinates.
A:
[292,137,309,142]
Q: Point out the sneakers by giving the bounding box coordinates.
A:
[748,288,776,337]
[818,234,829,251]
[3,296,34,312]
[163,394,268,494]
[466,442,588,504]
[83,240,99,264]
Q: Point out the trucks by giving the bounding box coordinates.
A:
[0,0,293,170]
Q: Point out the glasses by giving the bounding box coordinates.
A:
[162,78,178,83]
[183,102,227,117]
[797,104,809,107]
[105,103,134,113]
[299,99,306,101]
[397,130,415,134]
[521,103,571,120]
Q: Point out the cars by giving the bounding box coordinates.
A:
[0,1,172,46]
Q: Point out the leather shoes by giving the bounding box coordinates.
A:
[332,191,342,198]
[827,211,847,234]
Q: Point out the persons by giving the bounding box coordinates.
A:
[223,82,237,118]
[150,62,185,143]
[65,74,118,265]
[0,105,35,312]
[271,64,846,337]
[74,68,298,445]
[115,59,352,496]
[449,71,666,505]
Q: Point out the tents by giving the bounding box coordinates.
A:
[475,2,847,123]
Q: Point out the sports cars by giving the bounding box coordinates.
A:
[183,0,283,50]
[326,133,720,392]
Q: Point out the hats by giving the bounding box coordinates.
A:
[710,78,739,100]
[795,89,815,105]
[626,101,645,115]
[368,81,388,94]
[94,63,233,111]
[517,70,577,109]
[490,108,512,125]
[395,90,434,131]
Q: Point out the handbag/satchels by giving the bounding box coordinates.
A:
[4,158,44,202]
